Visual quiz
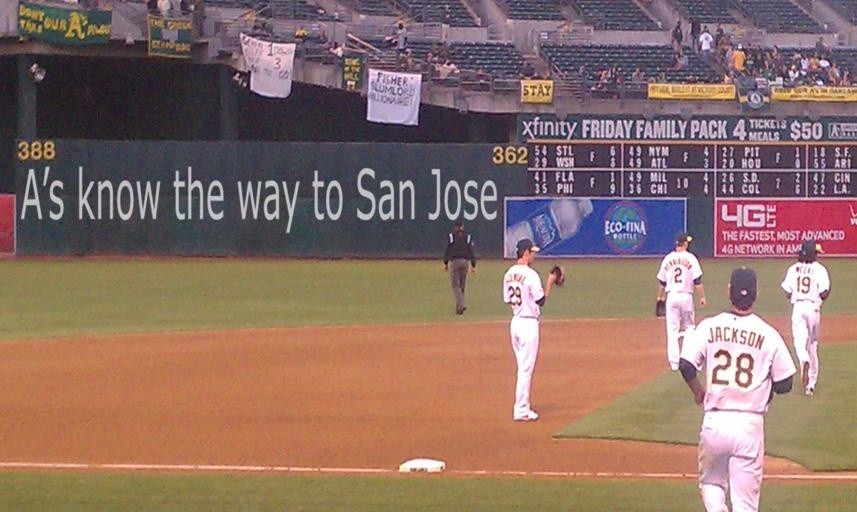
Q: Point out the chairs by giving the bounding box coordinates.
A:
[200,0,857,112]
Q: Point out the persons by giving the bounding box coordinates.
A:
[668,17,853,89]
[500,239,563,423]
[677,266,798,511]
[515,58,543,82]
[474,65,489,82]
[591,64,644,111]
[443,217,477,315]
[78,1,458,83]
[778,240,832,397]
[654,232,706,371]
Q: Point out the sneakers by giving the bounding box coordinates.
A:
[513,410,539,421]
[800,359,815,394]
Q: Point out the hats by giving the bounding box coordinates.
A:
[516,237,541,256]
[729,268,757,296]
[674,230,692,244]
[798,239,817,259]
